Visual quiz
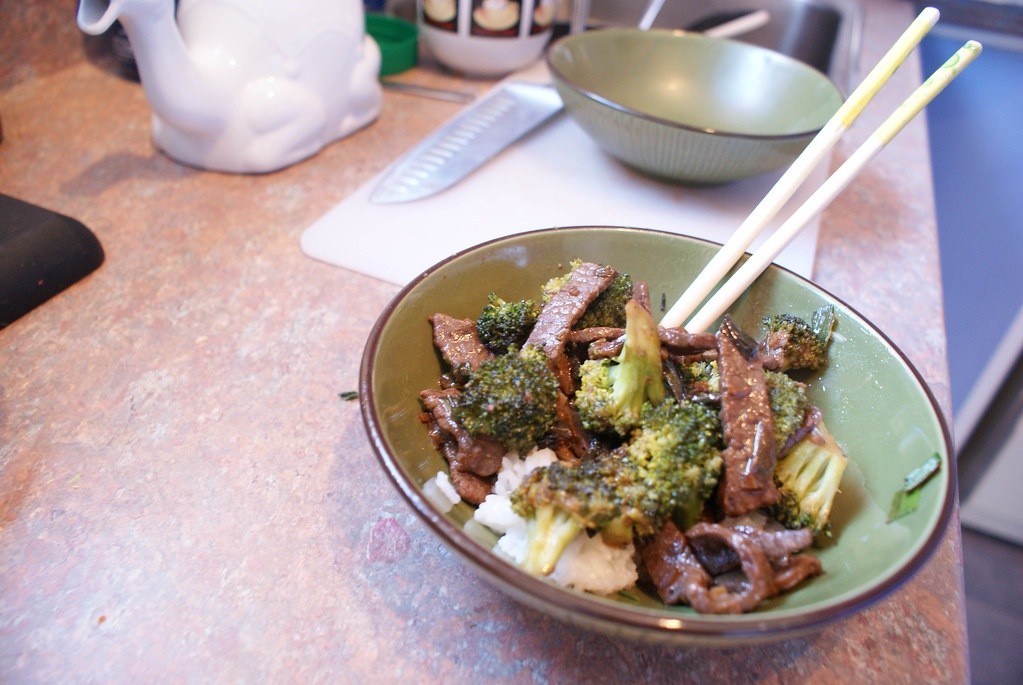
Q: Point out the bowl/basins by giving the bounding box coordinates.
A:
[545,28,848,185]
[358,225,958,649]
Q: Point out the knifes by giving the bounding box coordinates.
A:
[369,9,769,204]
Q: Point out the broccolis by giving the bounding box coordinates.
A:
[450,262,850,574]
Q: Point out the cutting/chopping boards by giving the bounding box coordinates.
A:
[303,62,835,287]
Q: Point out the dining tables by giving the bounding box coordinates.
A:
[0,0,969,685]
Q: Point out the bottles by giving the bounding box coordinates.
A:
[421,0,556,81]
